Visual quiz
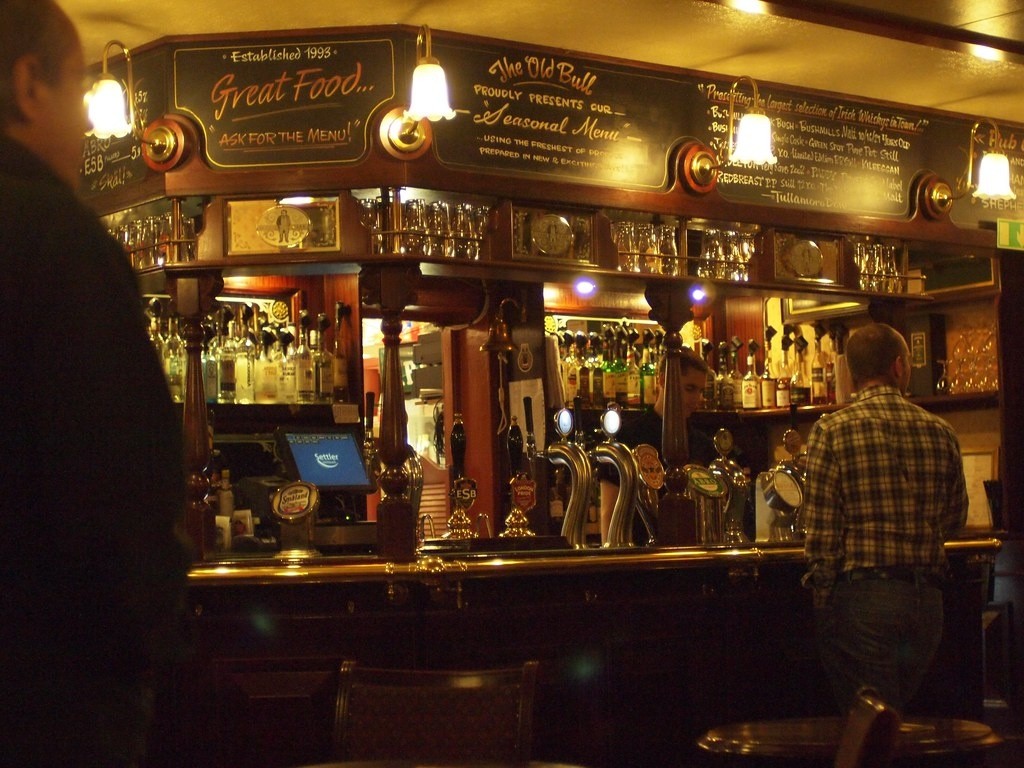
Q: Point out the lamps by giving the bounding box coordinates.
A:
[680,76,776,195]
[81,40,187,174]
[919,123,1014,223]
[380,24,459,163]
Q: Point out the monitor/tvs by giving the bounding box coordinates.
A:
[274,426,377,494]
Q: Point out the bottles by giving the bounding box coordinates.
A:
[703,325,836,409]
[360,195,492,259]
[108,211,196,269]
[851,242,903,293]
[611,219,678,274]
[552,318,667,410]
[149,297,349,405]
[696,227,756,281]
[207,468,234,527]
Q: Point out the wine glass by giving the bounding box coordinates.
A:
[935,320,997,392]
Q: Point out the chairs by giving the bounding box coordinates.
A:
[330,659,540,768]
[831,684,900,768]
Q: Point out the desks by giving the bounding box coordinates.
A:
[697,715,1004,768]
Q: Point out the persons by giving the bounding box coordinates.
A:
[803,323,968,716]
[0,0,189,768]
